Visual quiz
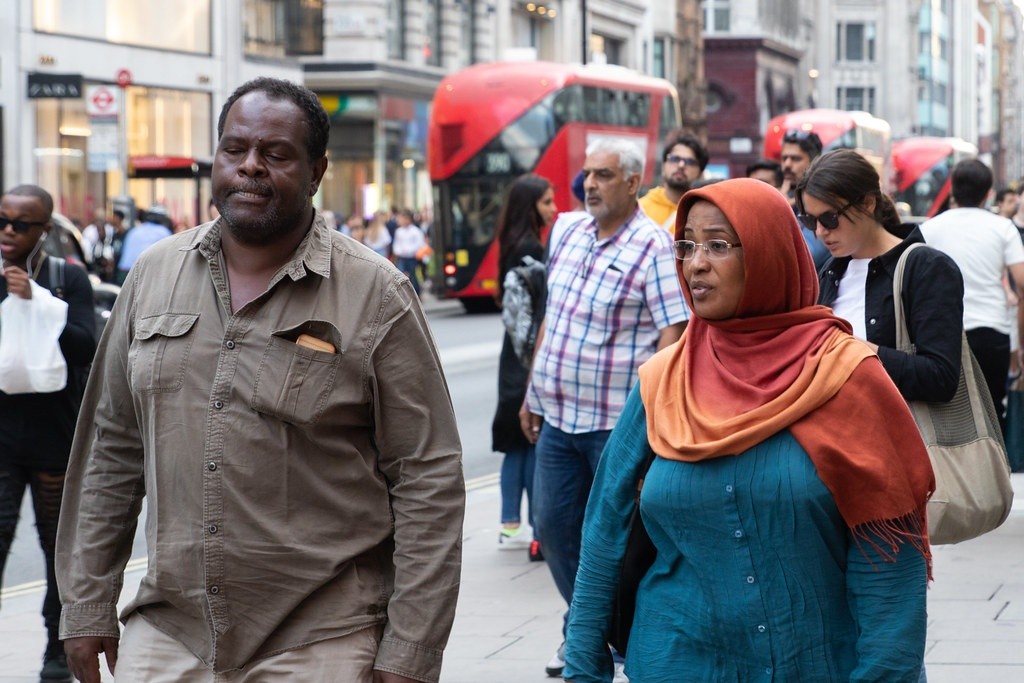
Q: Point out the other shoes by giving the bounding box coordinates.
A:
[546,641,568,675]
[39,632,75,683]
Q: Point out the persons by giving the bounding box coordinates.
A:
[72,199,220,287]
[493,128,1024,675]
[55,80,467,683]
[0,185,97,683]
[321,205,436,303]
[561,177,936,683]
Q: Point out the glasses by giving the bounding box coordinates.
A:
[797,200,860,230]
[0,218,44,233]
[671,238,742,263]
[783,129,811,139]
[666,154,699,166]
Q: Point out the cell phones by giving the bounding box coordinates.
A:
[296,334,335,354]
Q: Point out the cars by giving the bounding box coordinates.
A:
[39,212,123,352]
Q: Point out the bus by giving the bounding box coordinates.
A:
[761,105,892,194]
[887,135,980,219]
[423,54,685,314]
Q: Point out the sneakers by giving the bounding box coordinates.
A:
[498,522,534,549]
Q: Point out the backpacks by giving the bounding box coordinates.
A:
[499,254,546,366]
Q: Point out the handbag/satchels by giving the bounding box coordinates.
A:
[0,279,69,395]
[893,242,1014,546]
